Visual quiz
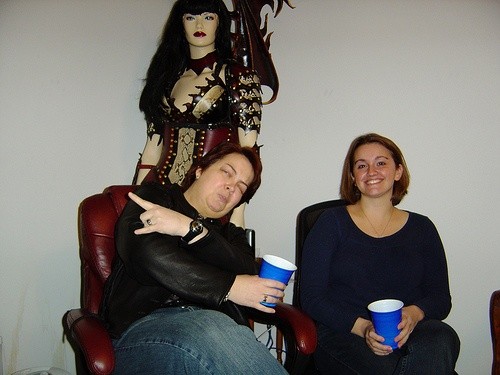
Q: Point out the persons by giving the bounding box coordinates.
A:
[136,0,263,231]
[98,142,291,375]
[298,134,461,375]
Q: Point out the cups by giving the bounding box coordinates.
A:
[368,299,404,350]
[259,255,297,307]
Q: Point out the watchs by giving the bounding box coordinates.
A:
[181,217,204,243]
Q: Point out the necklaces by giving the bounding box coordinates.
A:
[359,202,393,237]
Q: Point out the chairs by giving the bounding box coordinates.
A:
[62,185,318,375]
[284,199,351,375]
[489,289,500,375]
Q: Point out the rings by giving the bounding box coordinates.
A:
[262,295,267,303]
[146,219,152,226]
[410,330,412,333]
